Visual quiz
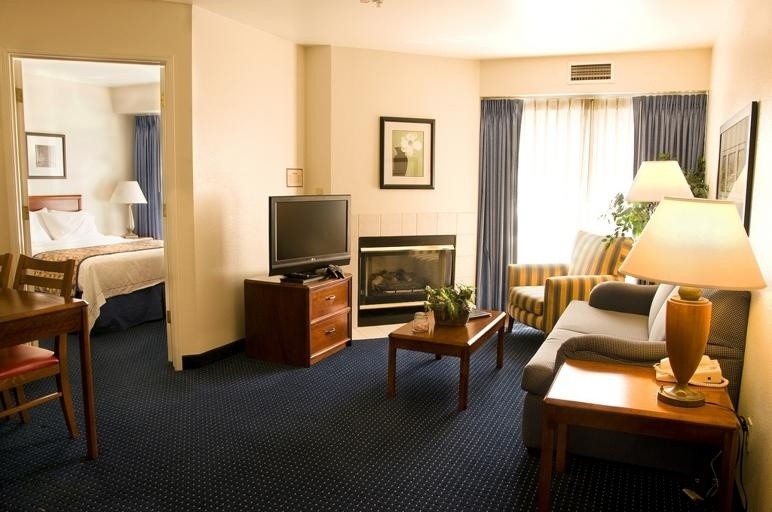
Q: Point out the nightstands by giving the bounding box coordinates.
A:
[135,236,153,240]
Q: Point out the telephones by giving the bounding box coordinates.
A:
[656,355,722,383]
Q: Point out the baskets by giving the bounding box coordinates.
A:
[435,305,472,326]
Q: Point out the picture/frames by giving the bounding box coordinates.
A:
[24,131,67,179]
[715,100,759,238]
[379,115,436,190]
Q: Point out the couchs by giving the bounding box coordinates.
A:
[519,280,753,482]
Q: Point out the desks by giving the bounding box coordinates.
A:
[0,285,99,459]
[542,357,742,511]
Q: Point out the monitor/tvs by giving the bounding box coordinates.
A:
[269,194,351,283]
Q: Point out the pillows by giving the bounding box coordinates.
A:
[28,206,101,245]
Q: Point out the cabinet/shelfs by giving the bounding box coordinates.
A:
[243,272,353,369]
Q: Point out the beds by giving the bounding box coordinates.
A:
[28,194,166,339]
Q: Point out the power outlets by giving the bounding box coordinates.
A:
[741,417,753,456]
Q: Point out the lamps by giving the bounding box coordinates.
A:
[617,196,768,408]
[626,159,696,203]
[108,180,148,240]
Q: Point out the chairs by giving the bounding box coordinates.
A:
[0,252,16,421]
[0,254,80,440]
[508,229,635,340]
